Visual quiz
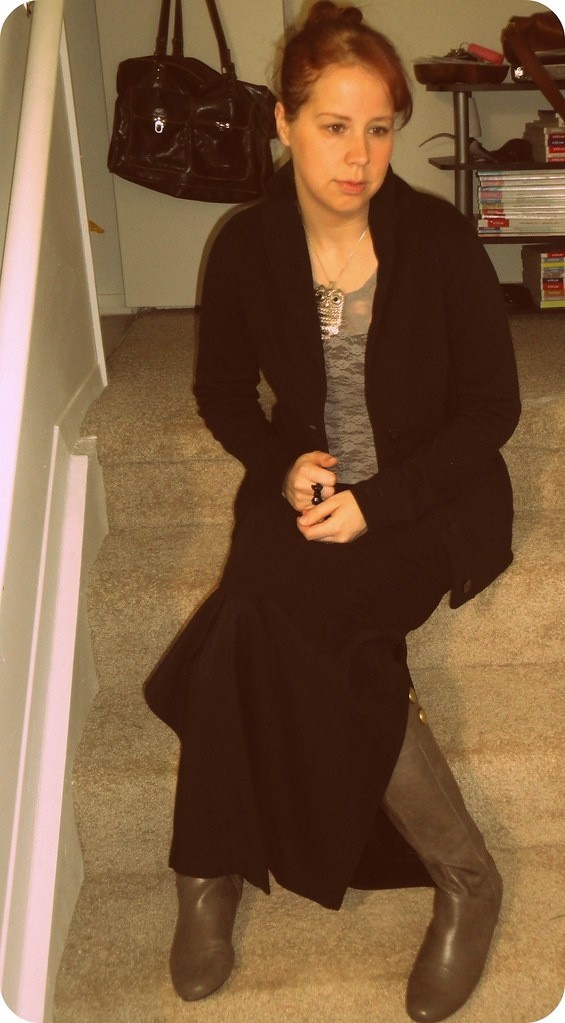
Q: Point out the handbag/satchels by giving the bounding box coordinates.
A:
[107,0,278,203]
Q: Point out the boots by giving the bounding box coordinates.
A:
[379,688,503,1023]
[169,869,244,1001]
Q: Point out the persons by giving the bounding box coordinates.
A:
[138,1,520,1022]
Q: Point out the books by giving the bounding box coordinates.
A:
[521,110,565,164]
[475,166,565,235]
[519,244,565,309]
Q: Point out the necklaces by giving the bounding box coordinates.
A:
[304,223,369,341]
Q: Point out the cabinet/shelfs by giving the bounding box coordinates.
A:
[426,83,565,315]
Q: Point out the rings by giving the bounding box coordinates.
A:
[311,483,323,505]
